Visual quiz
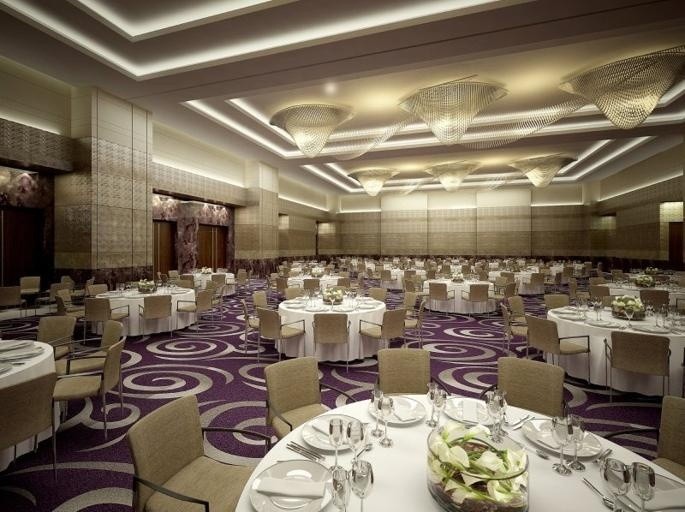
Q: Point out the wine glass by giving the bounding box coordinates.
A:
[552,411,584,478]
[575,294,681,332]
[330,418,371,512]
[369,389,394,450]
[488,390,511,435]
[426,382,445,431]
[602,457,655,512]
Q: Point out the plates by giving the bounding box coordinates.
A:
[551,306,589,321]
[522,417,601,458]
[371,396,424,427]
[251,413,365,512]
[282,296,382,312]
[444,397,504,426]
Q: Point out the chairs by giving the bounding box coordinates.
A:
[124,392,270,512]
[644,395,685,484]
[0,255,685,418]
[264,357,361,451]
[51,335,125,484]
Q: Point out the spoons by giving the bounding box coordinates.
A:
[582,477,617,509]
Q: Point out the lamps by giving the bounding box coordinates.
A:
[270,45,685,196]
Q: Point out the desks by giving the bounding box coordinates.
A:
[1,339,59,467]
[234,393,685,511]
